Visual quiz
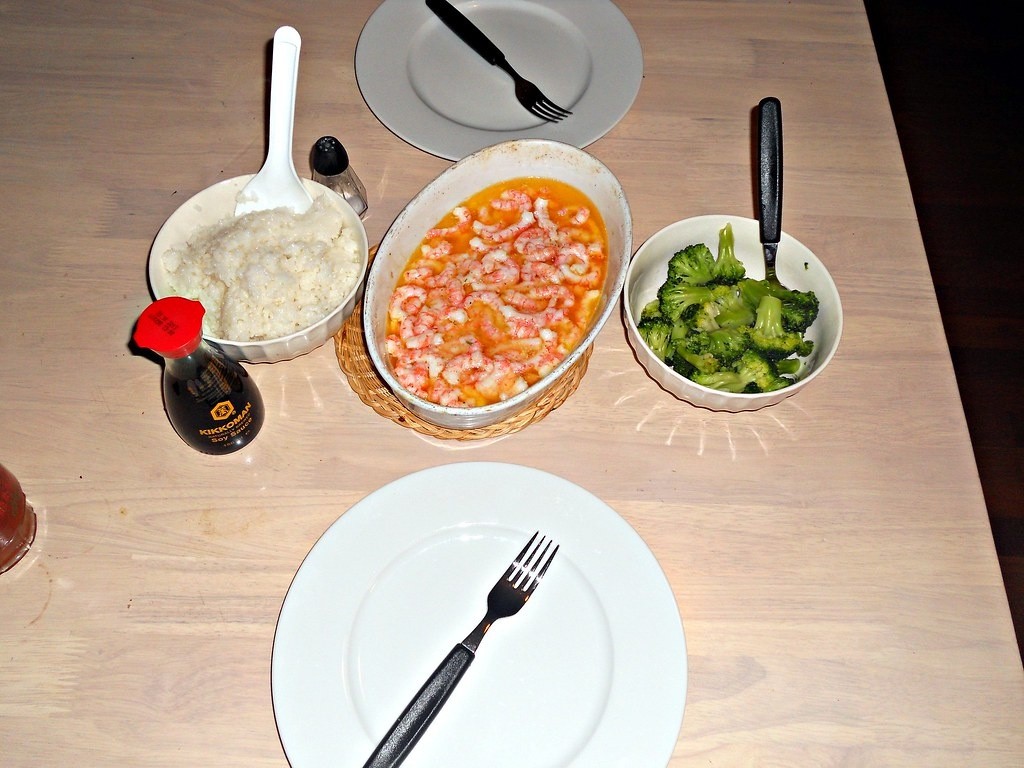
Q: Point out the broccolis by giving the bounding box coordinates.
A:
[637,223,820,394]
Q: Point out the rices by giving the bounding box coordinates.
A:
[158,192,362,342]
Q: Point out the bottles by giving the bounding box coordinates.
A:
[133,297,265,455]
[311,136,368,220]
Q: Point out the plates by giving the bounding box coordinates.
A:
[354,0,643,162]
[273,462,688,768]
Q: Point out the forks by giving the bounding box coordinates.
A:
[425,0,573,123]
[360,531,560,768]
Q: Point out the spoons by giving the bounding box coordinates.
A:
[233,25,313,219]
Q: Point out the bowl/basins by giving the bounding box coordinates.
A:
[624,214,842,413]
[149,172,370,365]
[363,140,633,430]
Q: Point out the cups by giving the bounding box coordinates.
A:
[0,461,38,577]
